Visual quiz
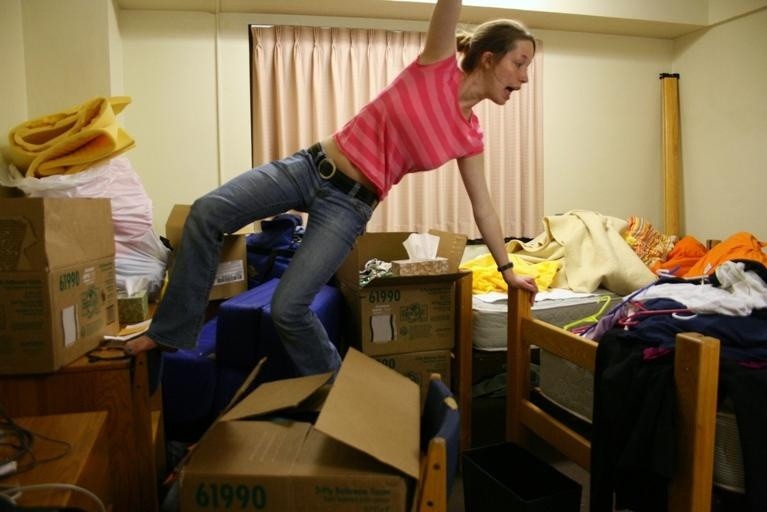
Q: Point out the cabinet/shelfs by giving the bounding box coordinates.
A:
[0,357,160,512]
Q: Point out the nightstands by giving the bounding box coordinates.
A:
[0,410,109,512]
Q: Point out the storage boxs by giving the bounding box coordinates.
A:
[179,346,421,512]
[0,198,120,377]
[165,204,255,301]
[370,351,452,407]
[336,229,469,356]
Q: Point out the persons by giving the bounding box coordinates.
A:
[125,0,538,382]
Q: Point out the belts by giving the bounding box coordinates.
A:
[303,142,385,212]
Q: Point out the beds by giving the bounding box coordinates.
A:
[451,239,723,452]
[503,283,749,512]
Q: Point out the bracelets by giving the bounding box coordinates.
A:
[497,262,514,271]
